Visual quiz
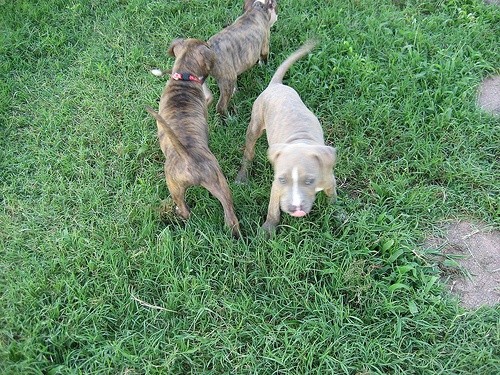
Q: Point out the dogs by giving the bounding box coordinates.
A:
[149,0,278,123]
[143,37,241,239]
[233,40,345,241]
